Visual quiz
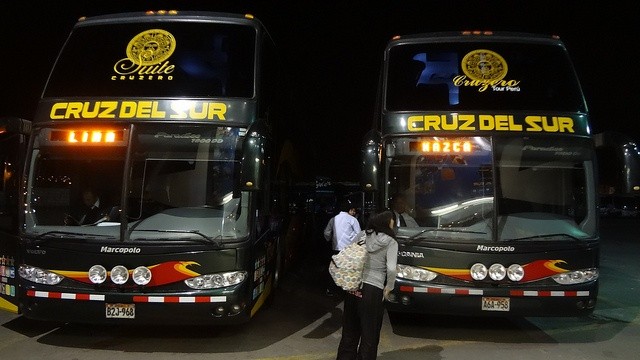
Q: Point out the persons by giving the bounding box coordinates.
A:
[64,189,112,226]
[319,203,361,296]
[336,208,399,360]
[389,193,419,227]
[346,205,364,230]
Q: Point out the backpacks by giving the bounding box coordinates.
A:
[329,231,367,291]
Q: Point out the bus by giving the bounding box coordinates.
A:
[12,16,276,327]
[361,29,636,324]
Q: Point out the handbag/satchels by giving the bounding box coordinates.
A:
[324,218,334,241]
